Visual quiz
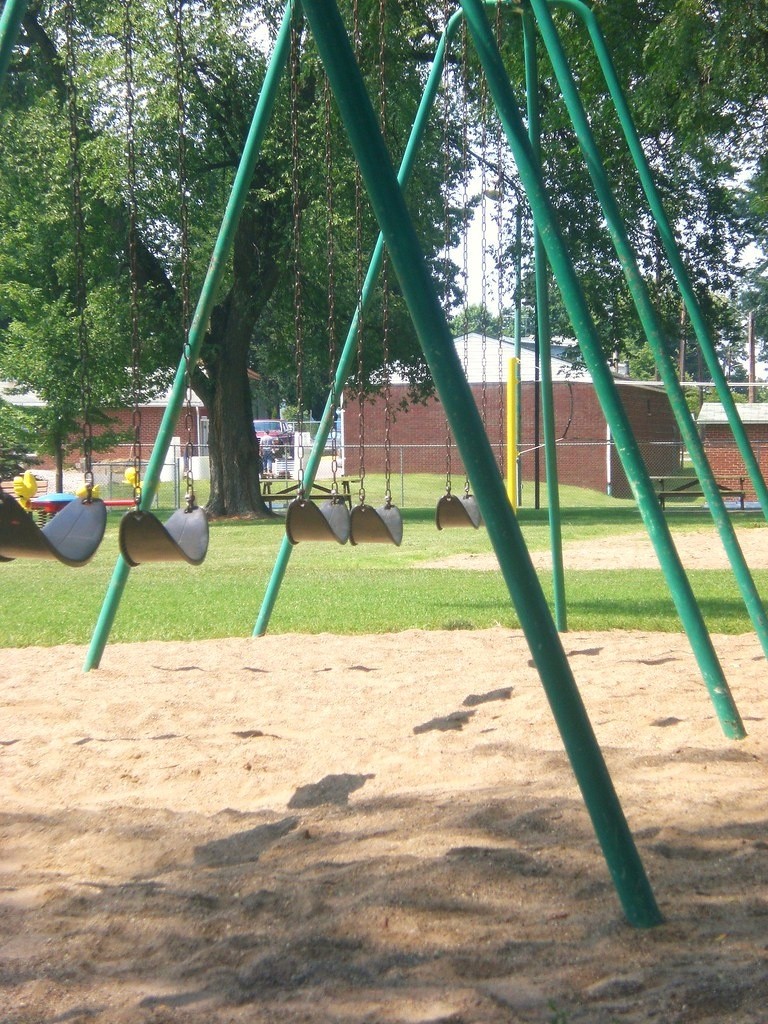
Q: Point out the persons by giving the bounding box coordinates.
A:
[259,430,275,474]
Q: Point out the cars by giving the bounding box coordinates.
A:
[252,416,294,460]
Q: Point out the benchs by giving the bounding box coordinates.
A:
[649,491,756,510]
[259,493,355,512]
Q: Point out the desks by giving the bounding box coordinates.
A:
[650,475,750,508]
[259,478,361,512]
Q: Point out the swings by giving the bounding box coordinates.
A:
[435,1,483,534]
[284,1,405,549]
[1,1,211,566]
